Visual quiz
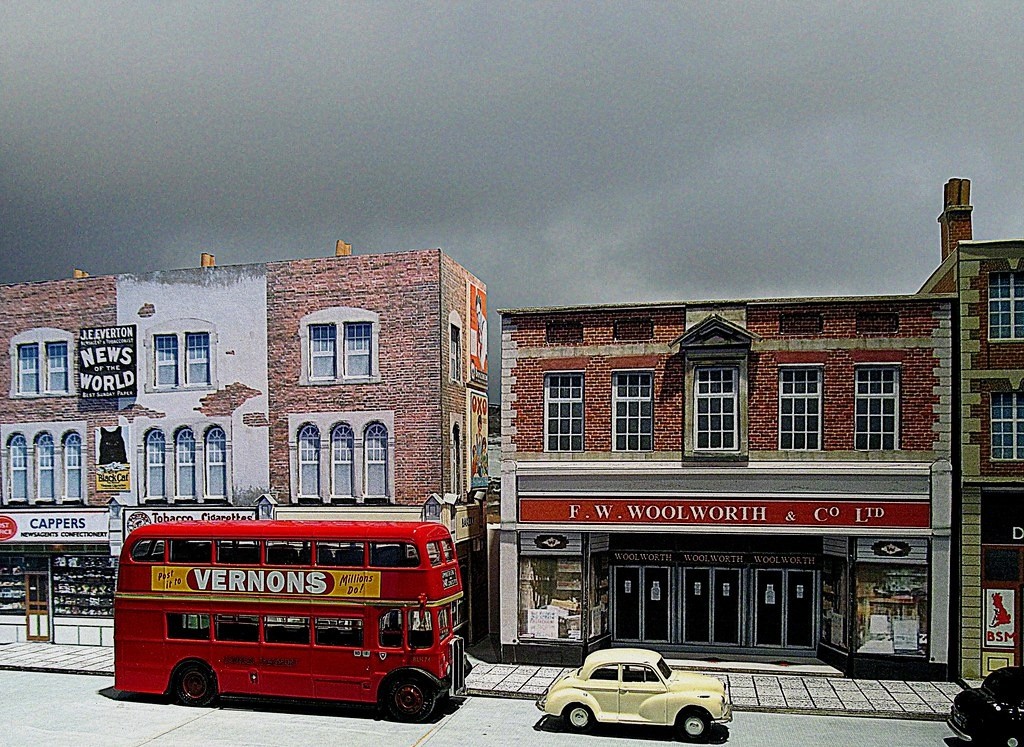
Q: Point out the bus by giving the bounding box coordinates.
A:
[113,520,473,724]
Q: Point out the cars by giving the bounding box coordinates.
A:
[536,649,733,743]
[947,666,1023,747]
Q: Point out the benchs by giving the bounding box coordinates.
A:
[172,542,211,562]
[375,546,403,566]
[382,630,403,646]
[218,622,256,642]
[292,625,309,643]
[325,627,339,645]
[299,544,333,564]
[219,544,260,563]
[267,626,289,641]
[268,544,298,565]
[324,546,363,565]
